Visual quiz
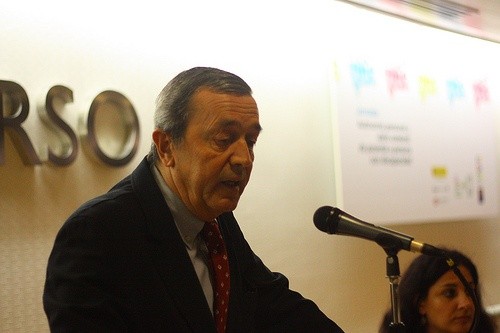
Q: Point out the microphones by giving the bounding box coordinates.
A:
[313,206,447,257]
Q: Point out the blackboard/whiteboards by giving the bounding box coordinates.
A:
[323,0,500,227]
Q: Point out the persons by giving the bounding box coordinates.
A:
[42,67,345,333]
[378,247,499,333]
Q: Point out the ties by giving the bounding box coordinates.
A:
[201,220,230,333]
